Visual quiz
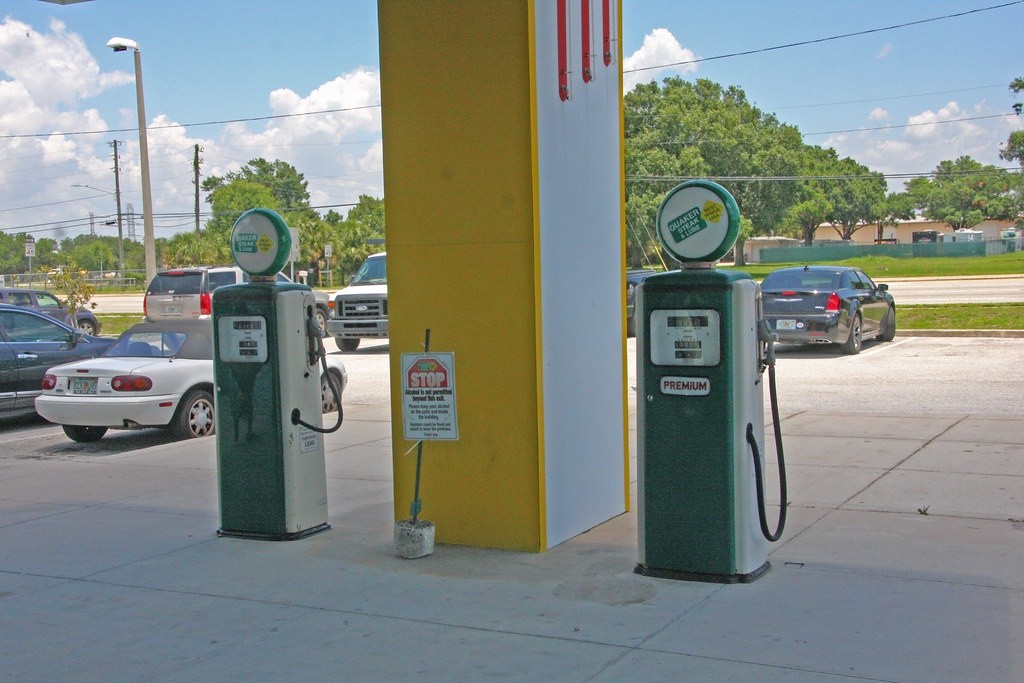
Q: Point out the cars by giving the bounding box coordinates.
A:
[0,286,101,342]
[34,312,218,446]
[756,264,895,355]
[625,269,657,337]
[0,304,145,426]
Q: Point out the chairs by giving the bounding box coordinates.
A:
[786,276,802,286]
[129,342,151,356]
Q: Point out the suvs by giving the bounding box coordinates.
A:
[143,264,331,342]
[326,252,389,351]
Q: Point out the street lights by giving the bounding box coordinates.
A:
[70,182,126,291]
[107,37,158,288]
